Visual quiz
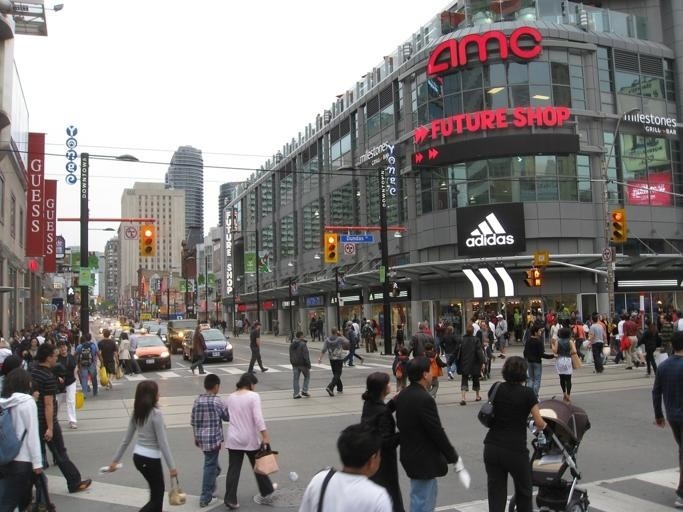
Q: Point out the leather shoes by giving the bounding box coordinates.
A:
[635,361,640,368]
[625,366,632,370]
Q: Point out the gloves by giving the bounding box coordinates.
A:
[453,456,465,472]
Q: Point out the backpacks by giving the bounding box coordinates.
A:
[78,344,92,367]
[0,409,21,465]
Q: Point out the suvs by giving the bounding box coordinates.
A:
[166,320,197,354]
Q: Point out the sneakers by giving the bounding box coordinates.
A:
[68,479,92,493]
[224,499,240,510]
[474,396,482,402]
[67,421,77,428]
[448,371,454,379]
[299,391,310,397]
[326,386,334,397]
[674,492,683,509]
[199,496,215,507]
[337,386,343,394]
[580,354,585,361]
[291,394,301,398]
[457,400,466,406]
[261,482,278,497]
[447,376,452,381]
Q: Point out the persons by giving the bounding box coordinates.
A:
[0,307,683,512]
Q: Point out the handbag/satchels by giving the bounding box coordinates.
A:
[98,365,108,387]
[573,332,579,339]
[168,484,186,507]
[475,401,495,427]
[253,440,279,475]
[503,332,511,340]
[454,358,462,376]
[570,352,582,369]
[74,391,85,410]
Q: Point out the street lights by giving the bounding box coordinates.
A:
[187,255,208,320]
[230,230,260,339]
[88,227,115,233]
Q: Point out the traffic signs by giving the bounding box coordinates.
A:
[340,236,375,243]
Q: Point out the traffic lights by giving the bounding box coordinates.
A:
[610,209,626,244]
[523,268,534,288]
[141,226,155,257]
[533,270,543,289]
[76,152,139,338]
[324,235,340,262]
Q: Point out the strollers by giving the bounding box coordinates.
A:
[508,399,591,512]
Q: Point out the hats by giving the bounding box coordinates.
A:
[345,320,353,326]
[365,319,371,324]
[495,314,503,319]
[252,321,263,326]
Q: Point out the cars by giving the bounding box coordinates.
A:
[182,323,232,363]
[127,334,171,370]
[88,312,169,346]
